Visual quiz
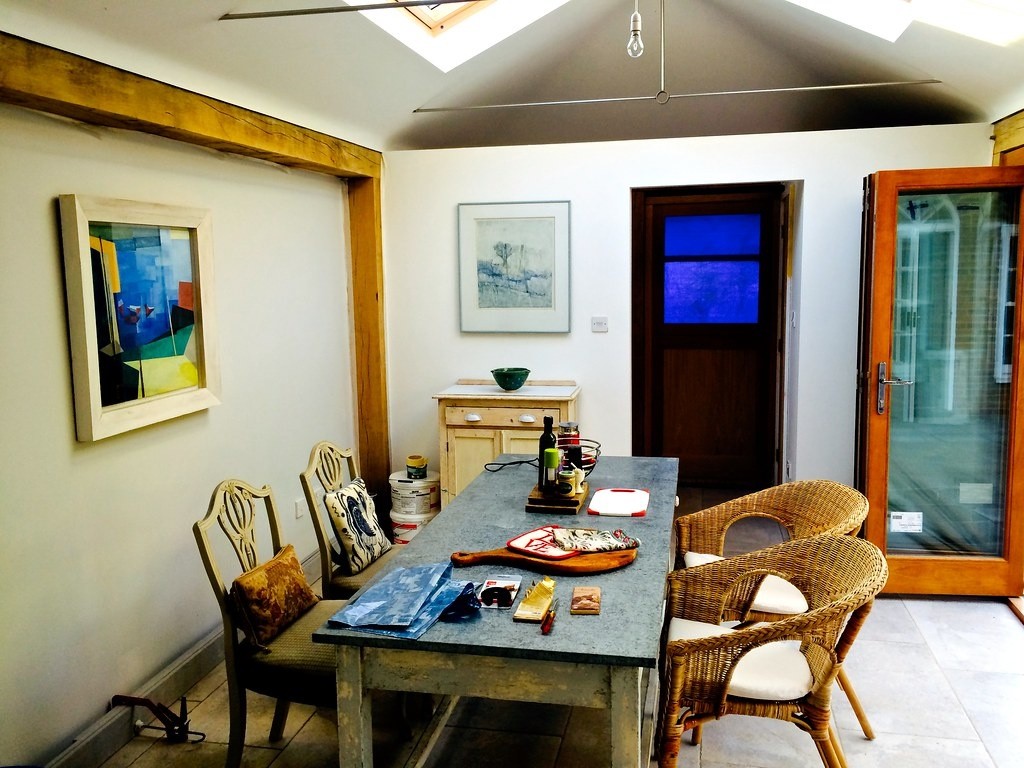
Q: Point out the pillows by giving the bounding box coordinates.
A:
[324,476,394,574]
[233,544,320,645]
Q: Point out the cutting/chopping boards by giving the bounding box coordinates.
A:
[452,529,636,571]
[588,488,649,517]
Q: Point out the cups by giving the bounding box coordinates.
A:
[556,422,580,465]
[559,471,575,497]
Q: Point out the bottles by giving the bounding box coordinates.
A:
[538,416,556,491]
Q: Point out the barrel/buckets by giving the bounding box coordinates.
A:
[389,470,440,514]
[390,509,440,544]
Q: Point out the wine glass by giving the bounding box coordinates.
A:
[573,469,585,493]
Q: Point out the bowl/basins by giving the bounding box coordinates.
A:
[491,367,530,390]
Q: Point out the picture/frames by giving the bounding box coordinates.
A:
[456,200,572,334]
[58,192,225,443]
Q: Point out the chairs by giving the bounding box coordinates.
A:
[191,440,408,768]
[656,477,889,768]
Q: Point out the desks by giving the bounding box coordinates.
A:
[310,454,679,768]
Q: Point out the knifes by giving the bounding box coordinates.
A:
[541,598,559,633]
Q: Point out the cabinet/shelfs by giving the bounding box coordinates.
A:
[431,376,583,512]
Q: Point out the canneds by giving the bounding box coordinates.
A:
[559,471,575,497]
